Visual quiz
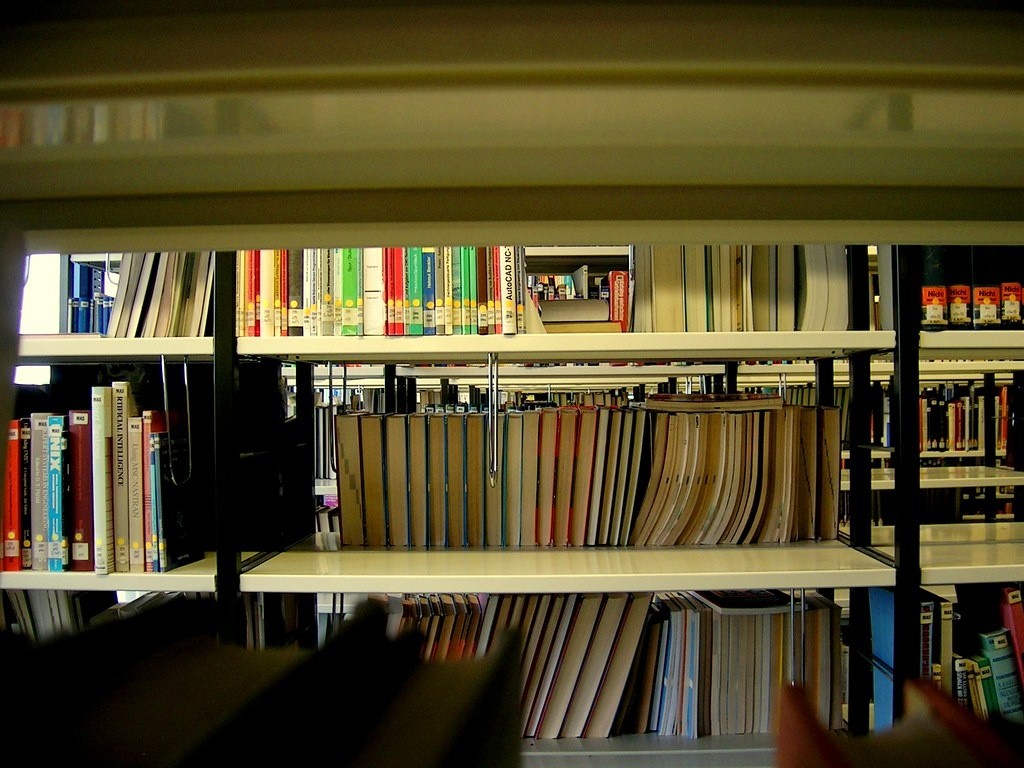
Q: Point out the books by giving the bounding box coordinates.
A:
[919,582,1024,720]
[884,384,1008,452]
[236,245,527,337]
[4,381,206,574]
[528,275,566,367]
[20,333,103,338]
[6,589,212,637]
[628,244,849,333]
[285,387,386,478]
[922,244,1024,332]
[368,589,843,740]
[104,251,215,337]
[538,270,629,366]
[334,389,842,547]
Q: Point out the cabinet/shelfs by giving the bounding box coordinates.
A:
[0,242,1024,768]
